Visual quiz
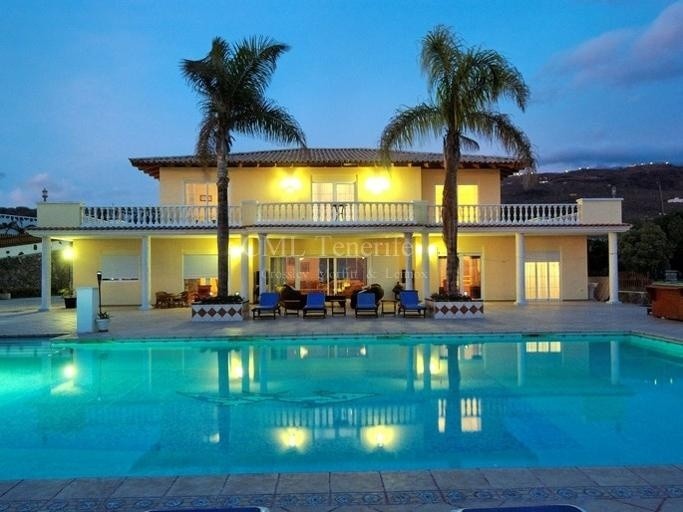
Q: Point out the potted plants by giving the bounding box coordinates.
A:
[60,289,77,308]
[96,312,112,332]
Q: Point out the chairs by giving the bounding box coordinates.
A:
[155,291,189,308]
[251,285,427,320]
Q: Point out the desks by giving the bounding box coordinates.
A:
[649,285,683,321]
